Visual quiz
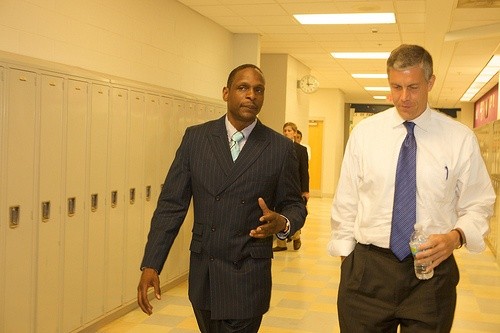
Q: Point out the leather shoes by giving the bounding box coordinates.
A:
[272,245,287,251]
[294,236,301,249]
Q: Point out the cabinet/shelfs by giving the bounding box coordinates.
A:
[0,50,227,333]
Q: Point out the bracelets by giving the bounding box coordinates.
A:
[451,229,463,250]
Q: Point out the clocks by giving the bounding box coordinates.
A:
[299,75,320,94]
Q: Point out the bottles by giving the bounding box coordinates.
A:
[410,223,434,279]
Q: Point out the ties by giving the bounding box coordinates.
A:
[229,130,245,162]
[390,120,417,261]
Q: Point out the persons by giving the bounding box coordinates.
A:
[331,44,495,332]
[273,122,311,252]
[137,64,308,333]
